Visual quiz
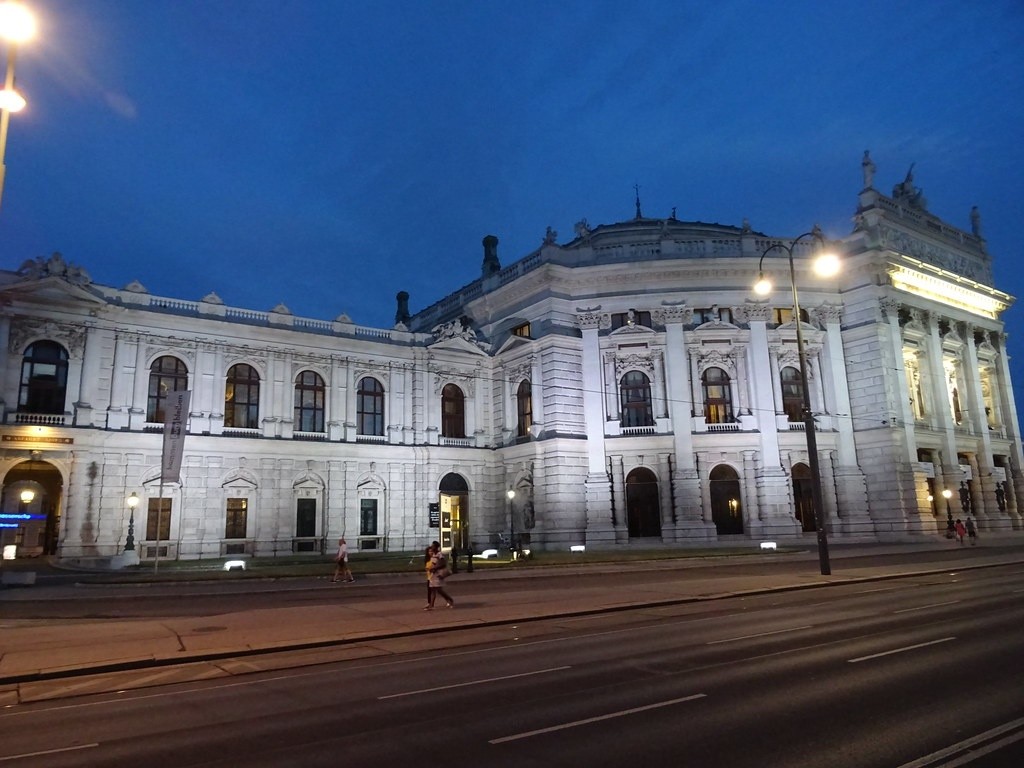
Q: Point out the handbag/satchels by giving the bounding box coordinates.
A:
[437,568,452,579]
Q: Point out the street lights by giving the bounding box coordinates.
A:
[0,1,38,201]
[507,484,515,552]
[756,233,839,576]
[14,486,34,571]
[942,490,955,539]
[124,491,140,551]
[929,495,933,513]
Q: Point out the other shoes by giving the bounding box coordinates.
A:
[446,601,455,609]
[423,604,430,608]
[423,607,435,610]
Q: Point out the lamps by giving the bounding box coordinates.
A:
[21,460,35,504]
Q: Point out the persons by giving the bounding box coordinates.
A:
[955,517,977,545]
[514,533,522,551]
[518,501,533,528]
[330,539,355,584]
[450,545,458,574]
[904,163,921,210]
[424,541,454,610]
[466,548,474,573]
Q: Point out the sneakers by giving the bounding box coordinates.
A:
[330,581,336,583]
[336,579,339,582]
[341,579,347,582]
[347,579,355,583]
